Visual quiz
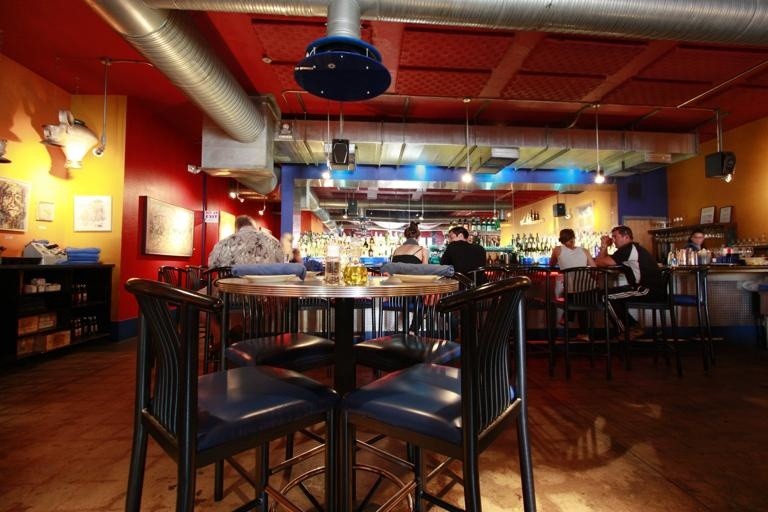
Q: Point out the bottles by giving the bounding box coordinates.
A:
[508,233,616,266]
[300,231,407,259]
[344,252,367,285]
[450,216,500,231]
[487,253,499,264]
[531,208,539,222]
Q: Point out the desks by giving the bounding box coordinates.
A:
[215,262,460,512]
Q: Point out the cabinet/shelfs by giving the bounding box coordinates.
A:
[647,219,727,263]
[0,263,118,377]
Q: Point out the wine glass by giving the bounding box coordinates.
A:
[654,216,724,242]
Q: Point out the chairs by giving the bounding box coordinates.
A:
[115,260,343,512]
[204,264,344,511]
[156,228,551,366]
[617,262,684,380]
[339,270,543,512]
[356,255,461,510]
[659,262,715,375]
[546,264,617,382]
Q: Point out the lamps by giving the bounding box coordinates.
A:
[88,57,115,159]
[256,194,267,216]
[462,95,476,188]
[186,163,202,176]
[492,189,498,221]
[342,188,348,219]
[236,181,244,204]
[419,190,425,223]
[590,99,605,186]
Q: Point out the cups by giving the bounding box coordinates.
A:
[323,256,342,284]
[669,247,754,266]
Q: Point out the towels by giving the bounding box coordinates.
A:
[62,247,101,265]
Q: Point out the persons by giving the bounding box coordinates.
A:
[549,229,598,341]
[440,226,489,341]
[195,216,285,350]
[683,228,706,251]
[594,225,663,341]
[392,221,429,337]
[289,248,301,263]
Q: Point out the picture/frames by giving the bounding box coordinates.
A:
[698,204,715,225]
[71,194,114,233]
[0,177,30,235]
[718,204,732,225]
[35,200,54,223]
[141,195,195,258]
